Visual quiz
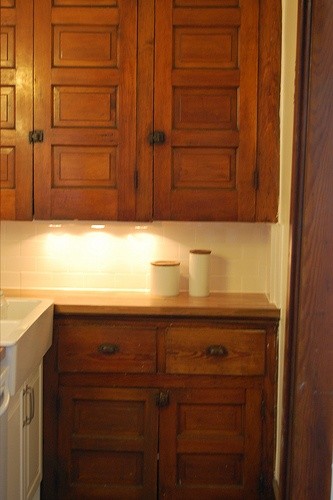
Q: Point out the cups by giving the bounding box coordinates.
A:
[188,249,212,297]
[150,261,181,296]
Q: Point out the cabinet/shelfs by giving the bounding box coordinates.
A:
[0,346,41,497]
[0,1,281,225]
[0,288,280,498]
